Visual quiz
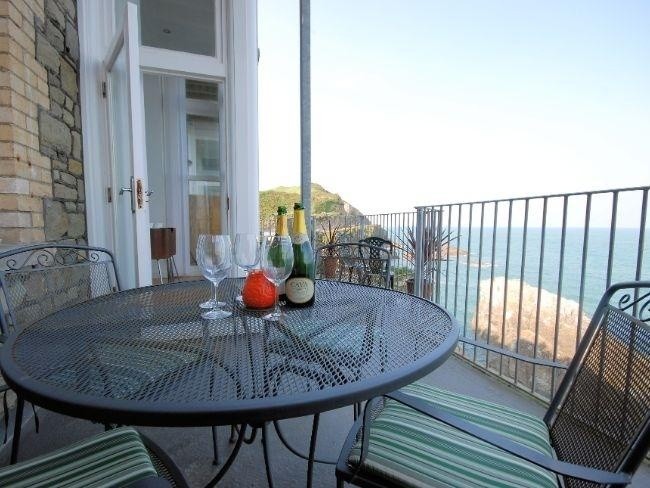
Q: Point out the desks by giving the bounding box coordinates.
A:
[149,226,183,284]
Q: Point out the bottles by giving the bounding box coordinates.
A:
[284,202,316,308]
[267,206,294,302]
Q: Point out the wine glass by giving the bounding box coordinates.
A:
[261,235,294,322]
[233,233,259,301]
[195,234,232,320]
